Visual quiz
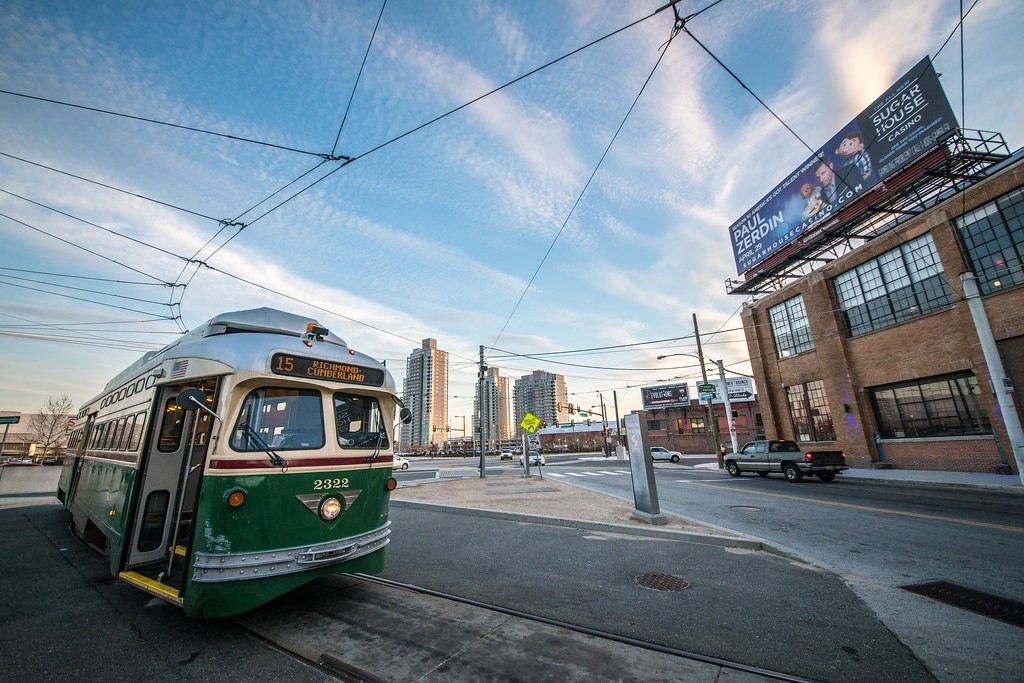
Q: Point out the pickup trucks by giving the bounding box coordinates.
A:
[722,438,849,484]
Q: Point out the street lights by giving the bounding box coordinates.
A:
[591,403,612,456]
[455,416,465,458]
[657,354,724,469]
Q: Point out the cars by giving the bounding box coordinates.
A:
[392,454,409,470]
[43,456,55,465]
[520,451,545,466]
[0,456,33,464]
[395,448,500,456]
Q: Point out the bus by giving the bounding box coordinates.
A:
[56,307,412,622]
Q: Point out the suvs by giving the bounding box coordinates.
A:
[649,446,682,464]
[501,449,512,461]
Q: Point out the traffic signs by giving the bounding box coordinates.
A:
[528,434,541,450]
[0,415,21,424]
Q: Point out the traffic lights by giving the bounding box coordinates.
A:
[557,401,562,413]
[543,421,547,428]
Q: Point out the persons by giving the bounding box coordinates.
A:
[835,130,872,182]
[520,445,523,455]
[606,434,612,456]
[493,449,496,458]
[813,157,866,210]
[720,441,727,462]
